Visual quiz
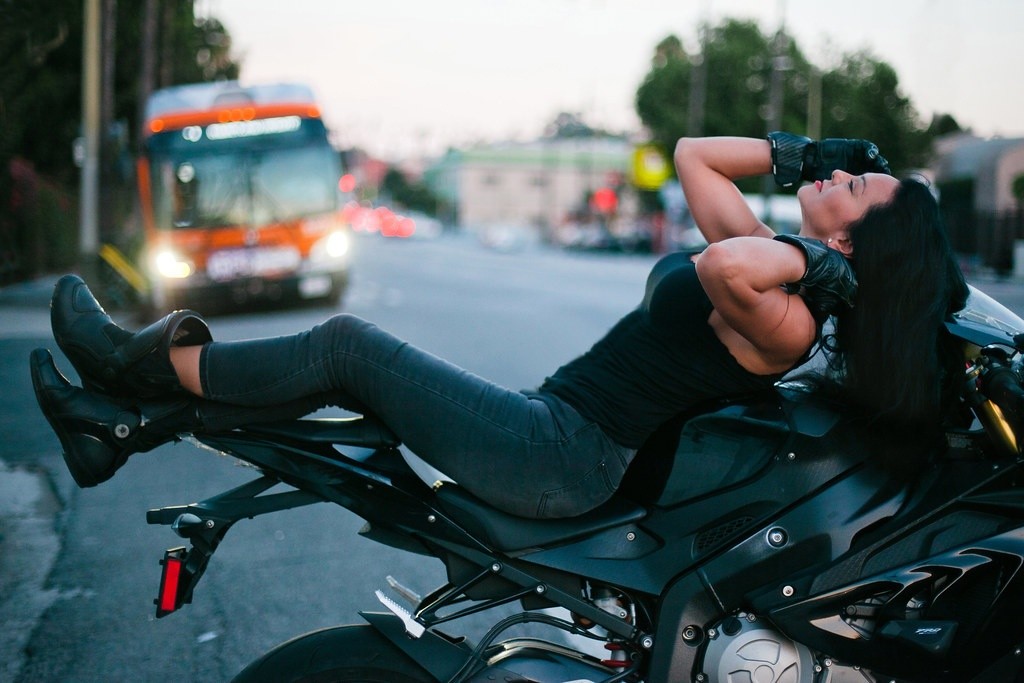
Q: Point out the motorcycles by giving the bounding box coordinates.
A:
[144,282,1024,683]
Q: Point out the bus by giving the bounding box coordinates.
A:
[138,77,355,323]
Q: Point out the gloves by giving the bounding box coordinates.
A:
[767,130,891,189]
[773,234,859,316]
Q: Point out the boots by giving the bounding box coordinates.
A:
[29,348,205,489]
[50,274,214,408]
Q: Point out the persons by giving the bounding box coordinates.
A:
[29,131,970,520]
